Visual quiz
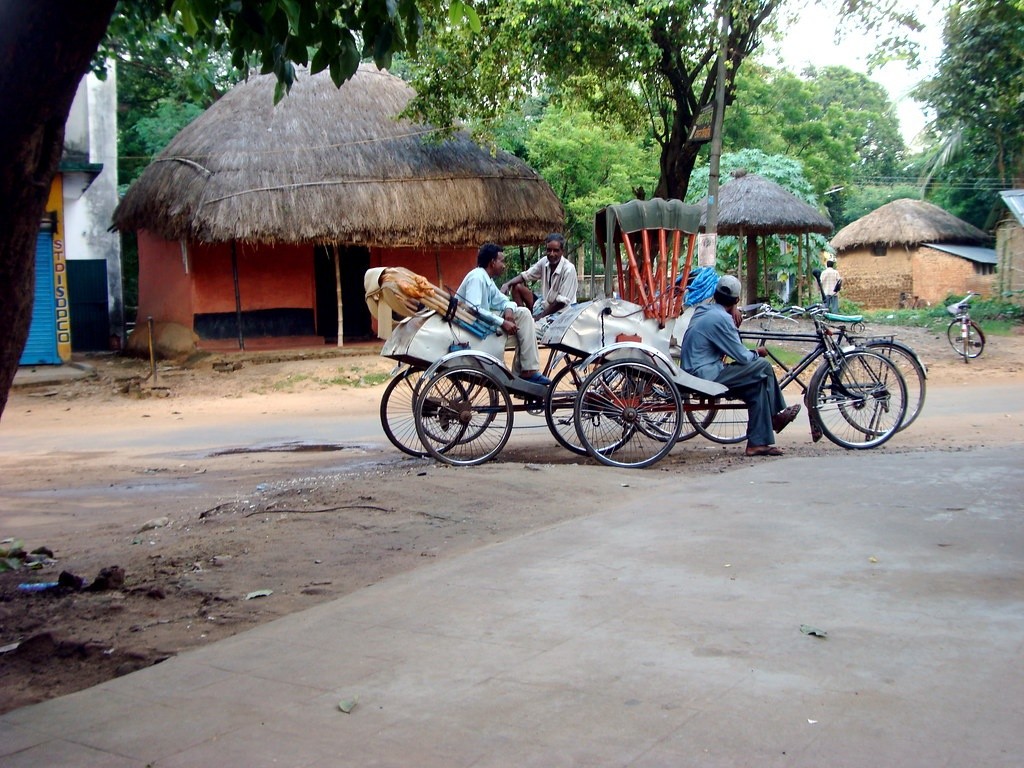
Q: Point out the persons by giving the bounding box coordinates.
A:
[454,243,552,385]
[818,260,840,320]
[680,275,801,456]
[501,233,578,340]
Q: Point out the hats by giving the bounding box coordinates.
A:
[716,275,741,297]
[827,258,836,263]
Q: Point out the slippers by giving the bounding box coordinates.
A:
[748,447,783,456]
[527,372,552,384]
[775,404,801,434]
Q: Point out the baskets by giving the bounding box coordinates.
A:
[947,302,962,318]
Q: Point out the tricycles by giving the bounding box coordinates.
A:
[363,197,930,471]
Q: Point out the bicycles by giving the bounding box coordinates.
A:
[947,289,985,366]
